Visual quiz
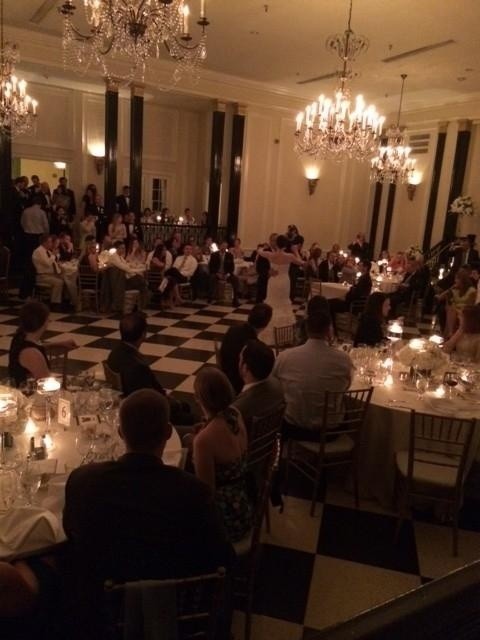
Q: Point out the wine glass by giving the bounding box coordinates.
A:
[0,371,121,506]
[350,344,480,400]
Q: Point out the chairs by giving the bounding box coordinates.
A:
[389,411,476,556]
[31,257,233,313]
[288,269,412,335]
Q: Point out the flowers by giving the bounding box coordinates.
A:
[448,195,472,217]
[397,343,447,372]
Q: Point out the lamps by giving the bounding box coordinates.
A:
[304,163,320,196]
[58,1,208,94]
[87,138,105,174]
[406,170,422,200]
[294,1,388,160]
[0,0,38,136]
[54,162,67,176]
[370,73,424,189]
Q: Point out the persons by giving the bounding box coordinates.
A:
[3,172,246,319]
[62,389,237,639]
[7,302,52,388]
[103,310,166,399]
[194,365,259,540]
[0,560,41,623]
[218,224,478,463]
